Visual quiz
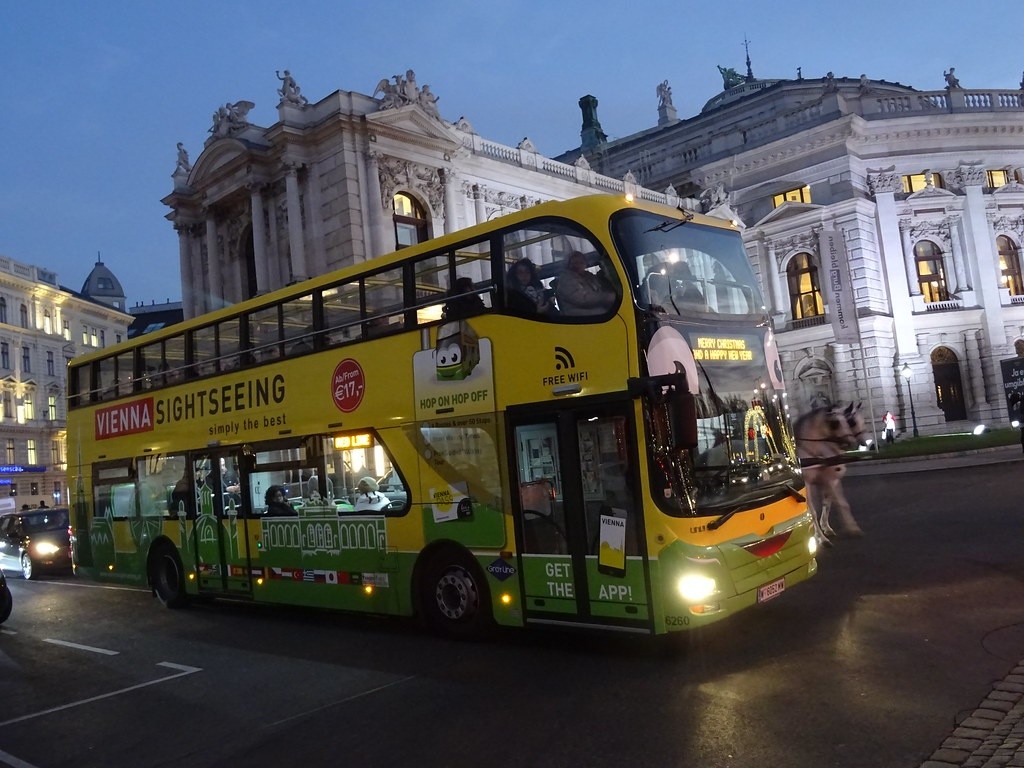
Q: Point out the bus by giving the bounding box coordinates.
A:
[64,193,821,643]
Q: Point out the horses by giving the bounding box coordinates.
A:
[792,400,873,550]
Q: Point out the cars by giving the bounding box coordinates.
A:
[0,506,70,579]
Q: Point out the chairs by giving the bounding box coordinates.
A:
[109,483,406,510]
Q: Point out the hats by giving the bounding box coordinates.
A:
[357,477,379,492]
[569,250,587,262]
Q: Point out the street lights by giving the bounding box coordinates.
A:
[901,362,920,437]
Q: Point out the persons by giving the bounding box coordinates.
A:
[882,411,897,446]
[275,69,301,107]
[555,249,615,317]
[373,62,447,125]
[206,102,244,138]
[366,308,391,333]
[220,458,240,503]
[504,257,553,314]
[455,277,488,314]
[264,484,299,516]
[171,142,192,177]
[354,476,392,512]
[658,82,672,105]
[38,501,49,508]
[943,67,962,89]
